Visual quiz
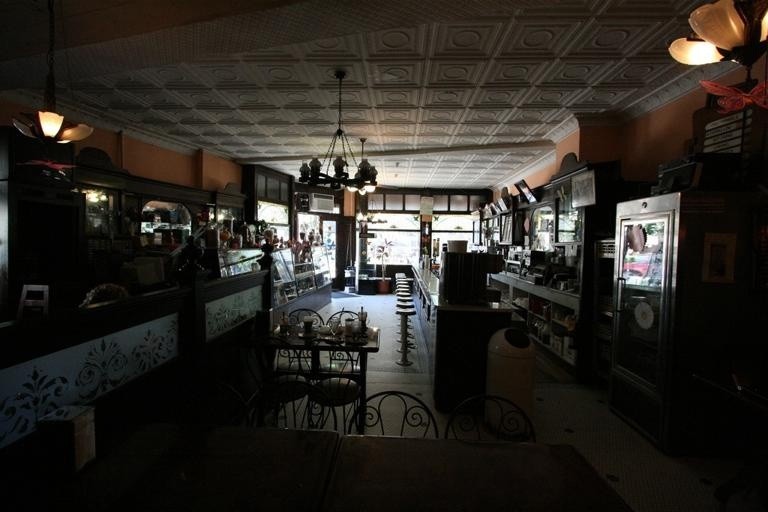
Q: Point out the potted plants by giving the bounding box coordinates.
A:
[376,238,394,293]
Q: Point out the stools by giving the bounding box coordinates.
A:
[395,272,418,366]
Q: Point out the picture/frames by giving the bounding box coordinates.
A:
[514,179,538,205]
[570,169,598,209]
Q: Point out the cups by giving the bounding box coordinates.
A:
[303,315,313,334]
[279,317,289,337]
[357,312,367,328]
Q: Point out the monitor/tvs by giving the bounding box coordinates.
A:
[571,172,597,208]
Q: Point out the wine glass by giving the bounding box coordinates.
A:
[328,316,340,340]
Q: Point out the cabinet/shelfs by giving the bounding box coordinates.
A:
[489,273,594,383]
[265,243,332,326]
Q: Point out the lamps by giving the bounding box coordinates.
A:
[10,2,94,147]
[667,1,767,82]
[298,67,379,193]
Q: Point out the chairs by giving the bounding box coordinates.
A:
[447,392,537,444]
[285,309,326,327]
[251,336,308,429]
[307,337,362,433]
[349,390,439,440]
[248,380,337,432]
[324,311,363,325]
[15,284,49,324]
[174,380,255,429]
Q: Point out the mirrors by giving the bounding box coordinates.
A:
[137,195,194,259]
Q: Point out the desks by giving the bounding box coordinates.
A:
[268,326,380,414]
[322,435,635,512]
[3,421,340,511]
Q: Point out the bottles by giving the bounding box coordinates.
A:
[345,318,354,337]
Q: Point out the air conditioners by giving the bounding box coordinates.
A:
[308,192,334,213]
